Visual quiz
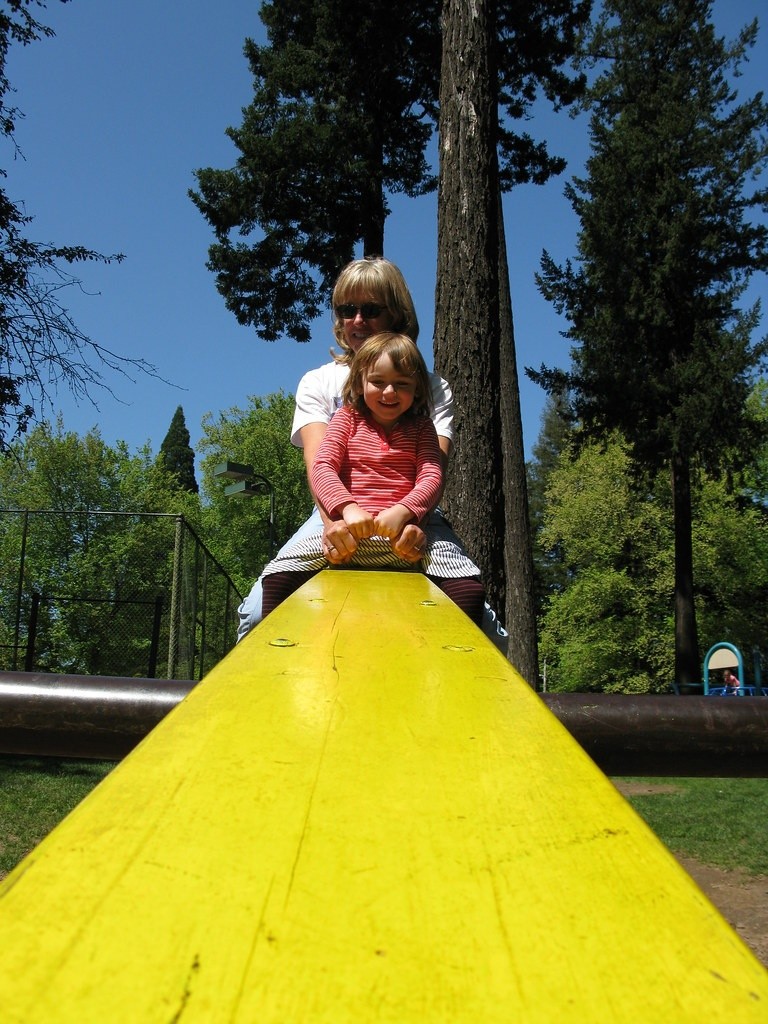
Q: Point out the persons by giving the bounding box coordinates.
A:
[235,257,510,659]
[723,670,740,696]
[261,332,487,630]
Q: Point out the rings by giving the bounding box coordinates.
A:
[413,545,421,551]
[328,545,335,551]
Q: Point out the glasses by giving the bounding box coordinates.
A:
[336,303,387,319]
